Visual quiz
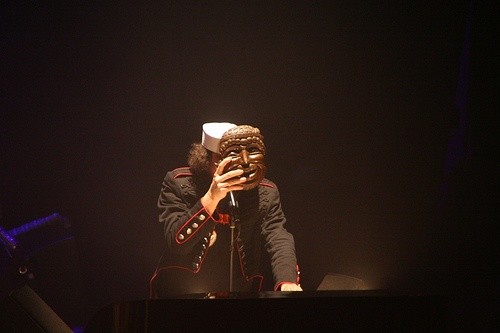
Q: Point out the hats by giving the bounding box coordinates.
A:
[202,122,238,154]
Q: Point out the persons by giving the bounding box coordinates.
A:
[147,122,303,300]
